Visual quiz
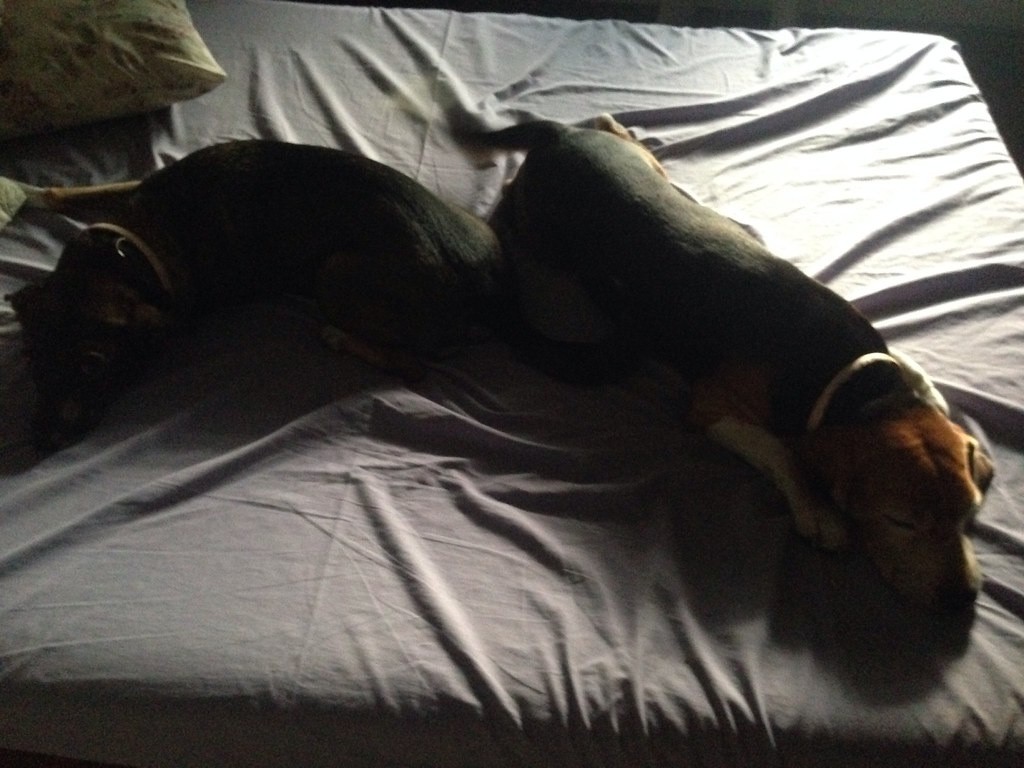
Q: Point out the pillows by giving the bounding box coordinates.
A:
[0,0,232,138]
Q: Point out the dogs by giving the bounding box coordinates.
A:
[5,140,638,455]
[444,103,998,617]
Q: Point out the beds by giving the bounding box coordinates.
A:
[0,0,1024,768]
